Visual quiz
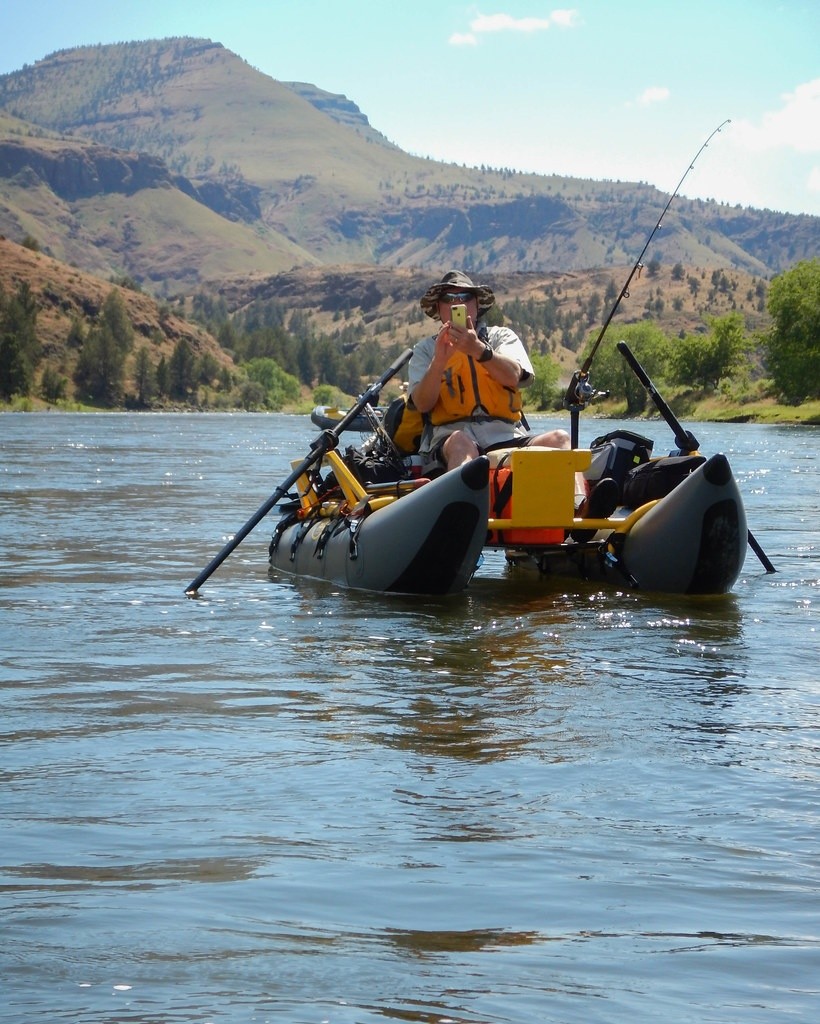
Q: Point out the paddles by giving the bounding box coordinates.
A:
[182,347,415,594]
[615,339,776,571]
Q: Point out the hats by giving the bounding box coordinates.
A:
[419,270,496,321]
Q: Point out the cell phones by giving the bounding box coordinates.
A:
[450,304,467,329]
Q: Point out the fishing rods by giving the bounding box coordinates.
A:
[579,118,733,383]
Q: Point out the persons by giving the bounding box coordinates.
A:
[362,383,379,412]
[398,382,409,404]
[408,270,620,545]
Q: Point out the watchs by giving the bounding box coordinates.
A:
[476,345,493,362]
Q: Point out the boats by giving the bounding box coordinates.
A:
[268,406,748,605]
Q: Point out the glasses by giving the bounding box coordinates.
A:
[440,291,473,304]
